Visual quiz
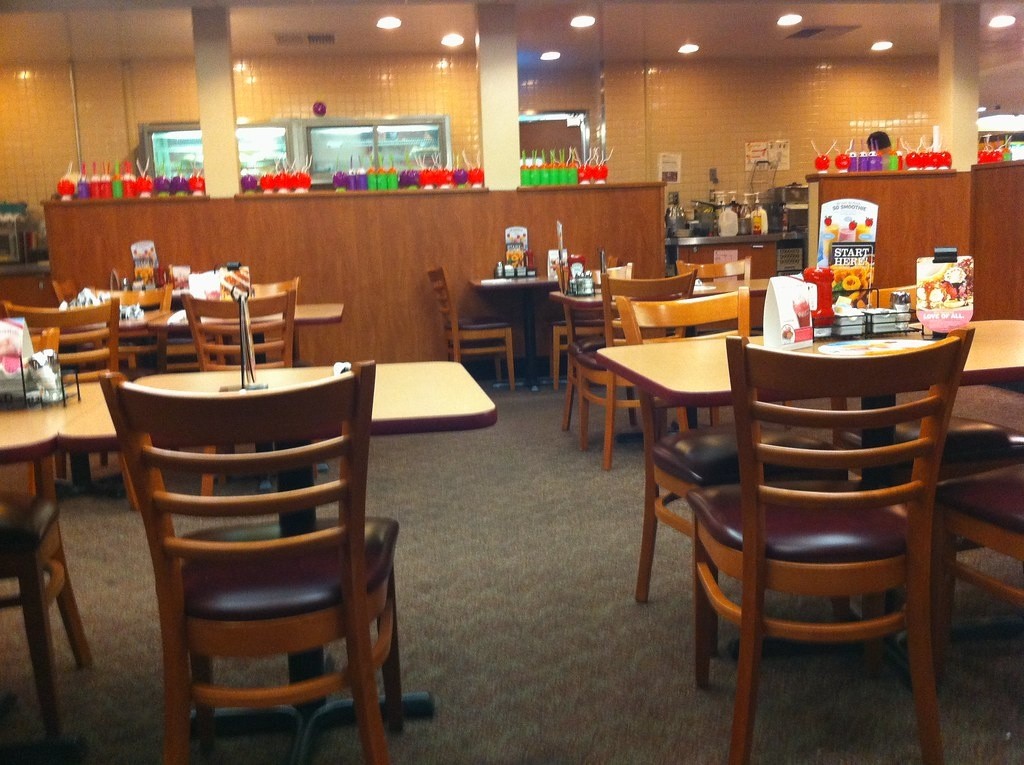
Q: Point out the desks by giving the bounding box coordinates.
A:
[598,320,1024,694]
[550,279,770,427]
[0,361,497,765]
[468,276,568,393]
[41,303,344,368]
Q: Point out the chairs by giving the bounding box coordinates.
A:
[686,325,975,765]
[676,257,751,280]
[562,262,637,431]
[90,283,172,368]
[428,267,516,393]
[1,299,119,383]
[616,287,848,602]
[0,493,94,745]
[575,267,698,471]
[99,360,403,765]
[182,290,295,370]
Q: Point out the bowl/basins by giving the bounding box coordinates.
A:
[665,207,687,237]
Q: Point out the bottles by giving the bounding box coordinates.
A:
[750,203,768,235]
[718,206,739,237]
